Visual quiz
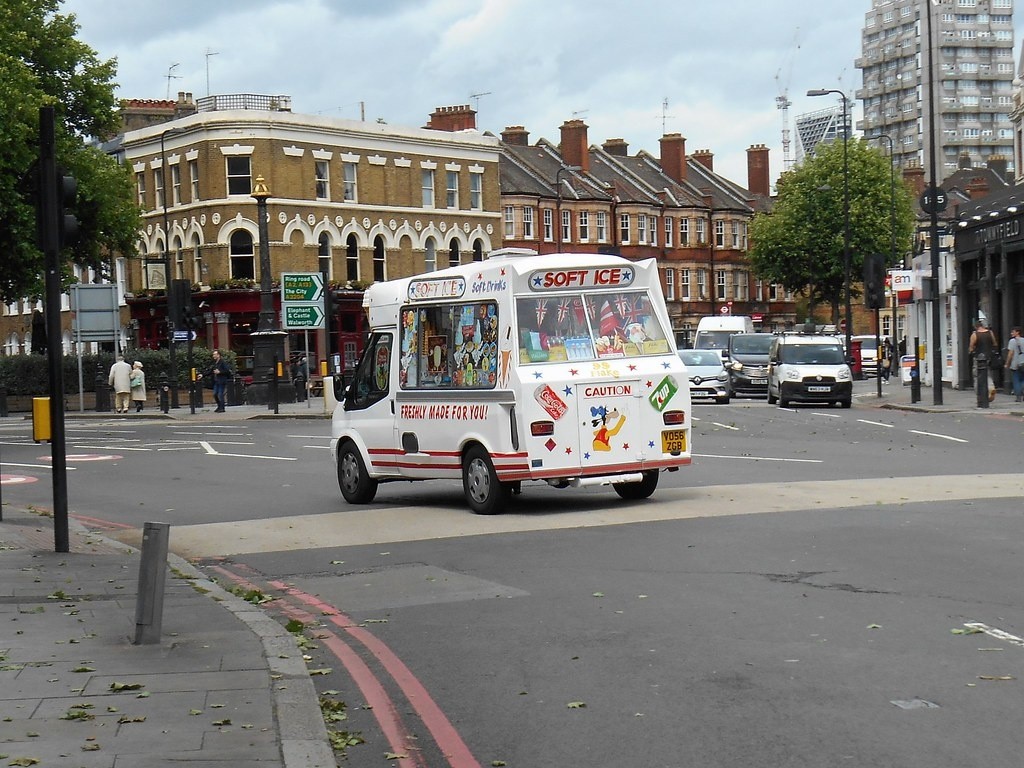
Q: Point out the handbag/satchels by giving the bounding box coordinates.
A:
[988,346,1006,371]
[883,359,889,368]
[1018,352,1024,367]
[131,372,141,388]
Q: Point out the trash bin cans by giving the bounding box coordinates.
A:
[323,376,345,414]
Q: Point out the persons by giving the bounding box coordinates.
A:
[969,321,999,402]
[211,350,229,412]
[897,335,906,357]
[1004,326,1024,402]
[129,361,146,411]
[107,356,133,412]
[880,338,893,385]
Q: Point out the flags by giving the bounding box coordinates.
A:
[618,296,644,330]
[599,300,620,335]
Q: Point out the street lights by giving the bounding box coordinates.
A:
[556,165,583,254]
[804,86,855,374]
[161,127,190,409]
[859,133,899,377]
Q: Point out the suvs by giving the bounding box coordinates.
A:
[765,330,853,409]
[721,332,778,398]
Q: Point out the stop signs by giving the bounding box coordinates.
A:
[840,319,847,331]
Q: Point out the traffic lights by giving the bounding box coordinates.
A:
[181,306,203,332]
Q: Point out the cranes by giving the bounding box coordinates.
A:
[770,24,803,171]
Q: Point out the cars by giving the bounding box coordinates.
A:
[676,349,733,405]
[828,333,894,377]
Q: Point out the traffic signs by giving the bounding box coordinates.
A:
[173,330,197,342]
[281,302,326,330]
[280,270,325,303]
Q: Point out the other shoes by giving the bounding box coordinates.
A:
[117,410,121,413]
[136,406,141,413]
[886,381,889,384]
[214,408,225,413]
[881,379,886,384]
[989,387,996,402]
[124,410,127,413]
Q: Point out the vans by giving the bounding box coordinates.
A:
[322,247,692,516]
[693,316,754,364]
